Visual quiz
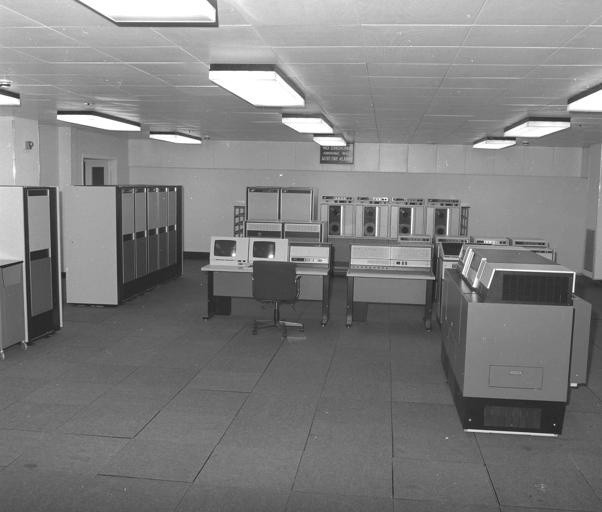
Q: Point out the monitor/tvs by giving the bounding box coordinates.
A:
[210,236,249,265]
[249,238,288,264]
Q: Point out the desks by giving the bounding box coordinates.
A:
[201,236,332,327]
[344,242,435,334]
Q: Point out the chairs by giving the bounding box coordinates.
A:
[250,258,306,340]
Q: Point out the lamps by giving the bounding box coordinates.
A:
[56,111,141,133]
[313,133,347,147]
[281,113,333,134]
[77,1,219,27]
[568,83,602,113]
[504,117,570,139]
[473,136,516,149]
[208,64,305,107]
[148,130,203,145]
[1,89,22,107]
